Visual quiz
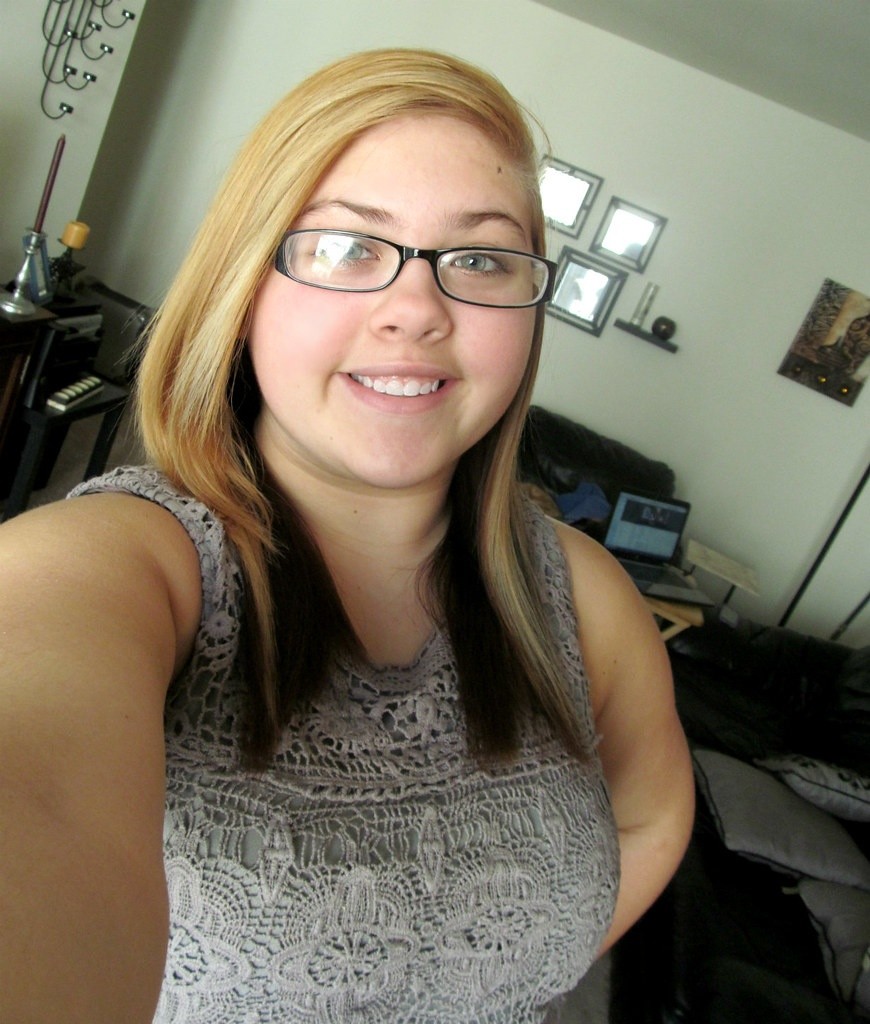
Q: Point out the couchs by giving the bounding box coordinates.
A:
[598,616,870,1024]
[527,405,677,522]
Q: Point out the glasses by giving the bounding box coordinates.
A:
[273,229,558,310]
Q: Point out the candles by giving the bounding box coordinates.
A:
[62,221,91,249]
[33,133,67,232]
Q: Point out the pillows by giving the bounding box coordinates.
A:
[692,749,870,892]
[753,753,870,823]
[797,876,870,1004]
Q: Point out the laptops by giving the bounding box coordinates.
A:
[601,487,716,608]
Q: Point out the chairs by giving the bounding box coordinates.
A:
[28,276,154,491]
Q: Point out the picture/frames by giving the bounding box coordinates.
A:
[544,245,629,338]
[586,195,667,273]
[537,154,605,240]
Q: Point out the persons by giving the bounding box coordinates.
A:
[1,46,698,1022]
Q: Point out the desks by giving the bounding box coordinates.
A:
[682,538,762,604]
[593,519,705,641]
